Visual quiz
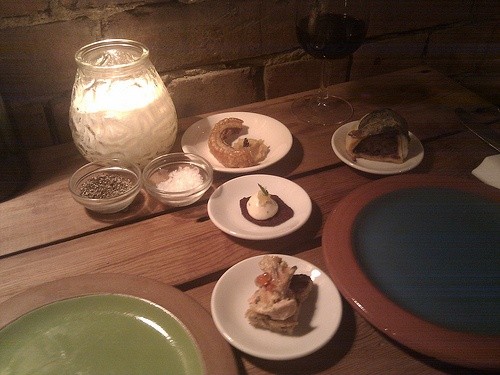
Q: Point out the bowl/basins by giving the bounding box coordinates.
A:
[142,152,213,208]
[68,159,142,213]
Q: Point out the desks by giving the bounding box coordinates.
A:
[0,67,500,375]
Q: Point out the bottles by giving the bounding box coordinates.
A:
[68,38,178,170]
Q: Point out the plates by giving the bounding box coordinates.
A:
[0,273,239,375]
[180,111,292,173]
[331,121,424,174]
[322,173,500,370]
[206,174,312,240]
[211,253,343,360]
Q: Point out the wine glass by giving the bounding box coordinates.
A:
[290,0,368,126]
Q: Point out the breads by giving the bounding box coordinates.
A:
[207,118,270,168]
[346,109,410,164]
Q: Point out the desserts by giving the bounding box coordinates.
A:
[246,255,312,333]
[239,183,294,226]
